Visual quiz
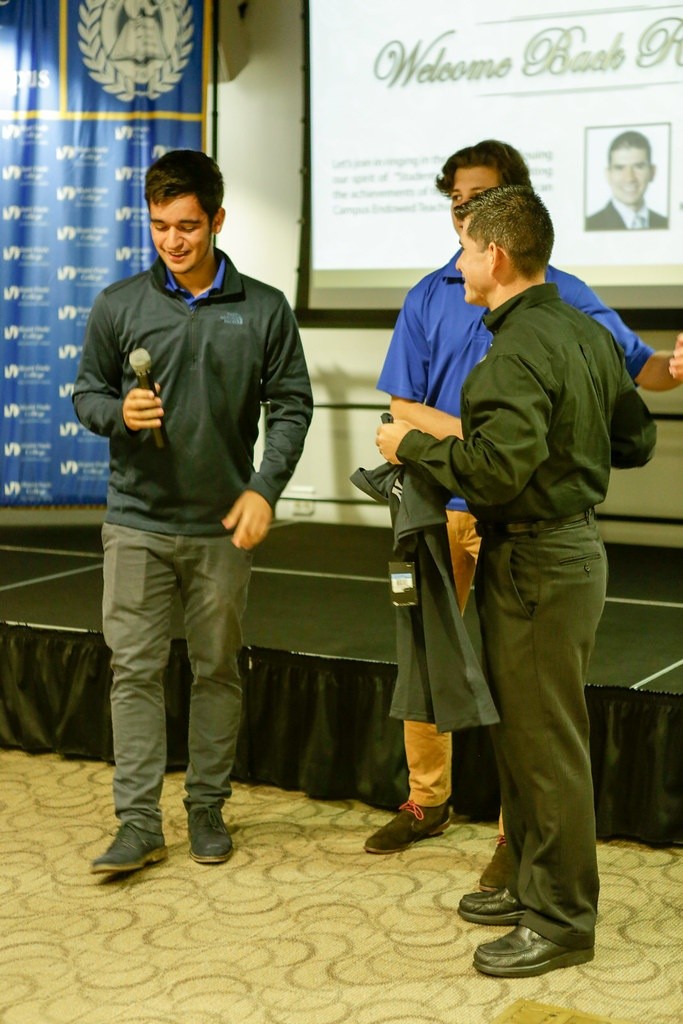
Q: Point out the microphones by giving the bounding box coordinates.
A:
[128,347,169,450]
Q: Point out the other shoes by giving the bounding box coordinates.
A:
[363,802,450,854]
[478,835,514,892]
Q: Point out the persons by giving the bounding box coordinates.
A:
[69,148,312,871]
[363,140,683,893]
[375,182,658,977]
[583,130,666,230]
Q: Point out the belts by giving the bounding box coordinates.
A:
[504,507,592,535]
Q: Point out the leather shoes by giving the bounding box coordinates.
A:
[473,920,595,979]
[186,806,234,864]
[457,885,527,926]
[89,822,168,873]
